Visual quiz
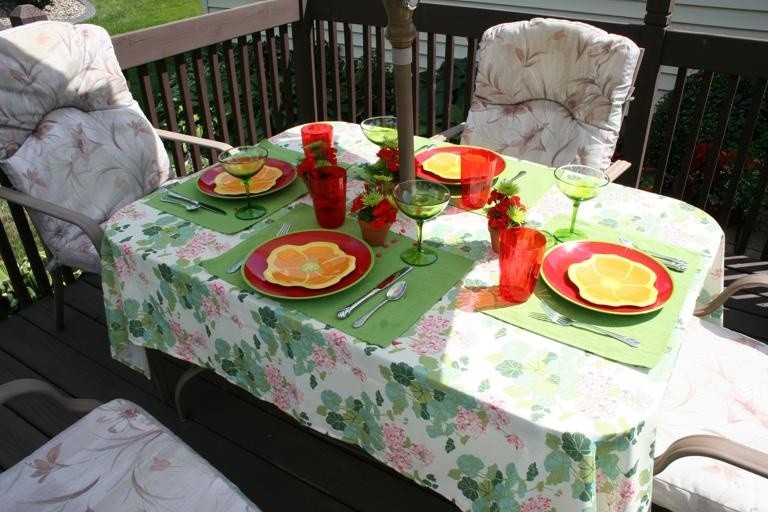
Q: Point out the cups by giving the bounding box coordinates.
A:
[306,166,347,229]
[460,149,498,208]
[498,227,546,305]
[301,122,333,157]
[448,285,528,314]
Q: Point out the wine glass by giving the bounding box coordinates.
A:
[392,179,451,267]
[217,145,268,220]
[554,164,610,242]
[360,116,399,183]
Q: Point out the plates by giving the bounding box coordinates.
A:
[541,240,675,317]
[241,228,375,300]
[196,156,298,200]
[414,145,507,186]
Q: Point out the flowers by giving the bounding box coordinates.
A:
[484,178,526,230]
[295,135,421,228]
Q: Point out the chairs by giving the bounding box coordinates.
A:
[0,20,245,406]
[0,372,263,512]
[647,270,768,511]
[430,13,647,189]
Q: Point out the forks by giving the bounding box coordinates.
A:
[225,222,291,274]
[544,305,643,349]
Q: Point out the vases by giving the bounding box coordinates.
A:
[355,217,391,247]
[488,226,505,255]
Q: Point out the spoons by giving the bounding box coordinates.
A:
[616,234,687,266]
[351,280,406,328]
[160,196,200,212]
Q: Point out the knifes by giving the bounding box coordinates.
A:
[337,265,412,319]
[168,190,227,215]
